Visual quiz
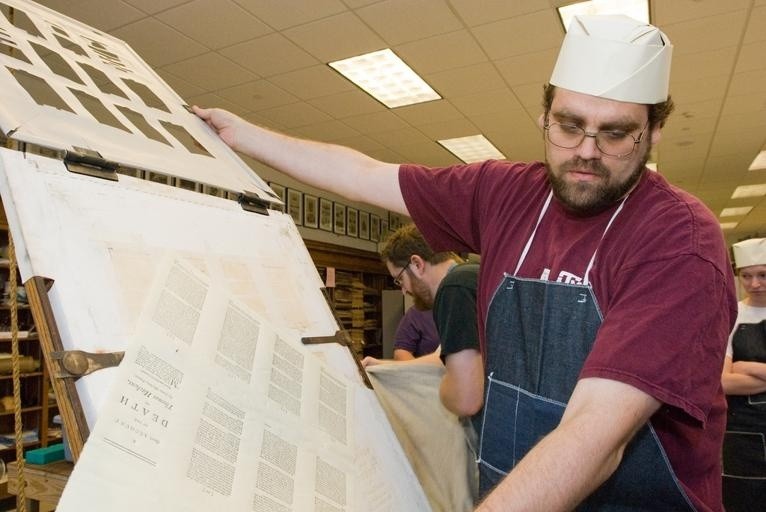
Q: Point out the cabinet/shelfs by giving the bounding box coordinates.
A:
[316,266,404,361]
[0,199,63,501]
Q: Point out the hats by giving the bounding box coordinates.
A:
[732,237,765,268]
[548,14,675,105]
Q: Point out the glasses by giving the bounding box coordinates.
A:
[544,113,650,158]
[393,260,412,287]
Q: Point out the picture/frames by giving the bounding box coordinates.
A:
[267,182,288,213]
[346,206,359,237]
[227,190,240,200]
[203,184,227,199]
[22,141,61,160]
[388,212,399,232]
[320,198,334,232]
[333,202,347,236]
[142,171,176,187]
[175,178,203,192]
[304,192,319,229]
[116,168,144,176]
[381,219,389,236]
[358,210,371,240]
[370,213,381,242]
[287,187,304,225]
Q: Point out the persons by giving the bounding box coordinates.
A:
[369,220,492,418]
[188,17,742,510]
[717,226,765,510]
